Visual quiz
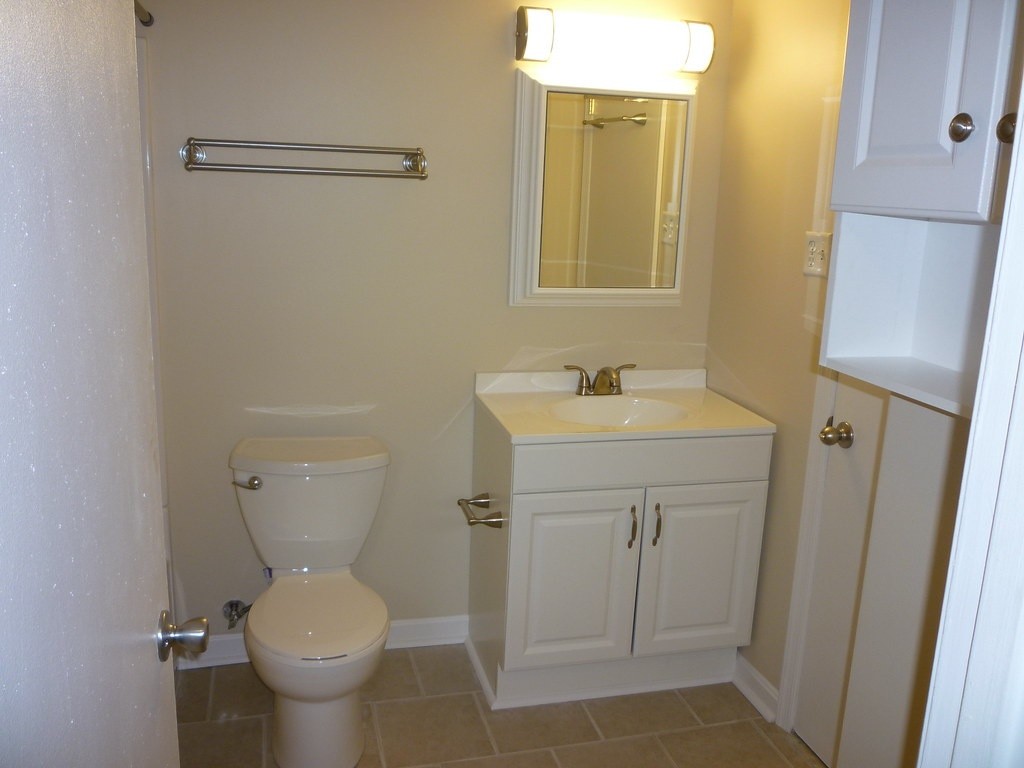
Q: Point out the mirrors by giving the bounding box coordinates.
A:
[507,66,699,309]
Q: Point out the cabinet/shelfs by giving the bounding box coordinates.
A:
[503,433,773,672]
[791,373,973,768]
[830,0,1023,227]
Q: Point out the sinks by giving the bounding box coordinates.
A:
[550,394,690,426]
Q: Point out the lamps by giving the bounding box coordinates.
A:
[515,5,716,74]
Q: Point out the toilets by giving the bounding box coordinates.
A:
[227,435,393,768]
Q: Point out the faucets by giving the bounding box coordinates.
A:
[592,366,622,395]
